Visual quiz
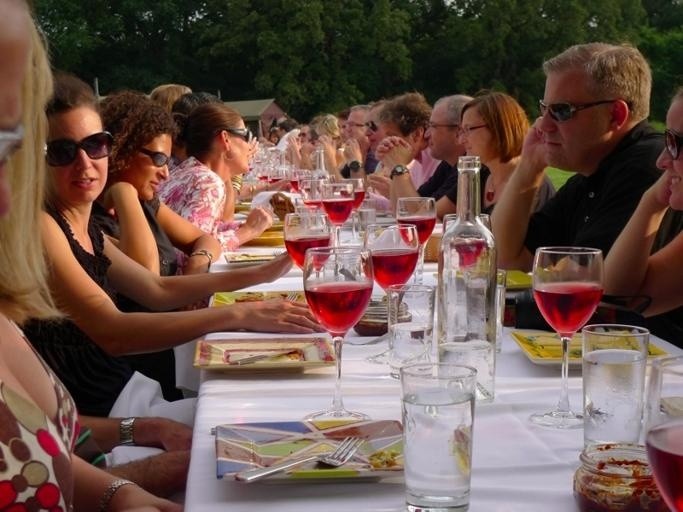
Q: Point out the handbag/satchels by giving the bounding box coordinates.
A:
[514,288,651,336]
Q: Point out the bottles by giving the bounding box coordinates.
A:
[436,155,500,405]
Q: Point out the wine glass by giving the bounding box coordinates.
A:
[300,244,375,425]
[244,145,439,337]
[532,245,603,431]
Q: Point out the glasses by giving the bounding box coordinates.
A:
[424,121,456,130]
[457,124,486,138]
[46,131,115,167]
[663,128,682,160]
[538,99,632,123]
[365,120,377,132]
[139,148,170,167]
[298,133,306,137]
[0,121,28,165]
[224,126,250,143]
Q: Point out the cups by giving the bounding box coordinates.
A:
[642,356,683,512]
[580,324,650,446]
[440,212,492,270]
[386,283,435,379]
[399,361,475,512]
[466,269,507,354]
[574,442,667,512]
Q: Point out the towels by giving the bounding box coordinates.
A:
[95,369,196,470]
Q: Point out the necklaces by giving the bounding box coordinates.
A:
[485,168,510,204]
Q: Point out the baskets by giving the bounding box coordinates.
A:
[271,193,295,221]
[422,233,442,263]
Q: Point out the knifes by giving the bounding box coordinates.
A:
[230,347,296,367]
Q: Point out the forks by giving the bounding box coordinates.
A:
[236,433,365,485]
[285,292,299,303]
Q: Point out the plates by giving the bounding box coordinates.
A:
[432,269,534,294]
[195,334,339,376]
[214,421,411,485]
[234,205,249,213]
[511,330,667,368]
[224,253,275,264]
[210,290,308,309]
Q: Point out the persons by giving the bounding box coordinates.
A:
[488,41,683,350]
[457,92,557,214]
[601,85,683,318]
[375,94,496,217]
[265,105,375,194]
[1,2,326,512]
